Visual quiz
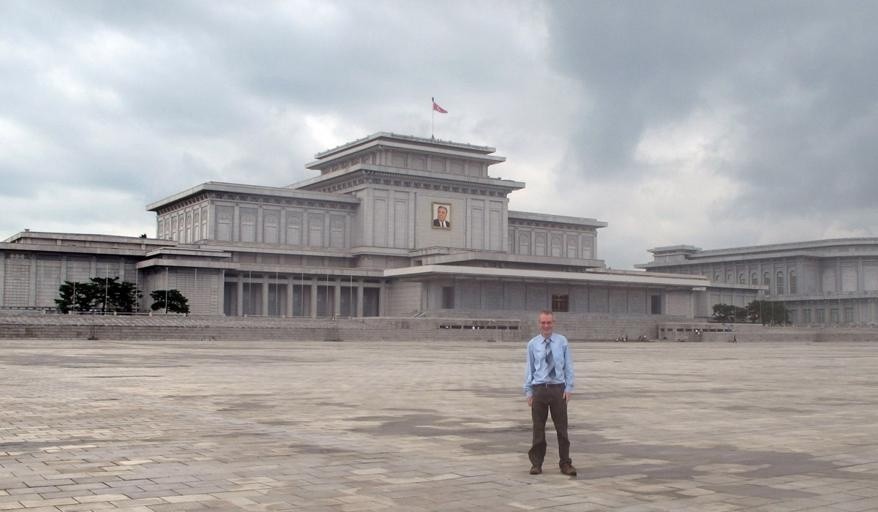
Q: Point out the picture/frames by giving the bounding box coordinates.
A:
[431,201,452,231]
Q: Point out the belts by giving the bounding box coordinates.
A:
[532,383,565,387]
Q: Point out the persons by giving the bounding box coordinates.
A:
[524,310,578,475]
[433,206,449,227]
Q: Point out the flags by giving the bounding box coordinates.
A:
[432,101,448,113]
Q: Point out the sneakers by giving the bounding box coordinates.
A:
[560,463,576,475]
[529,464,542,474]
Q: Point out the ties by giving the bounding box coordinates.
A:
[544,338,556,379]
